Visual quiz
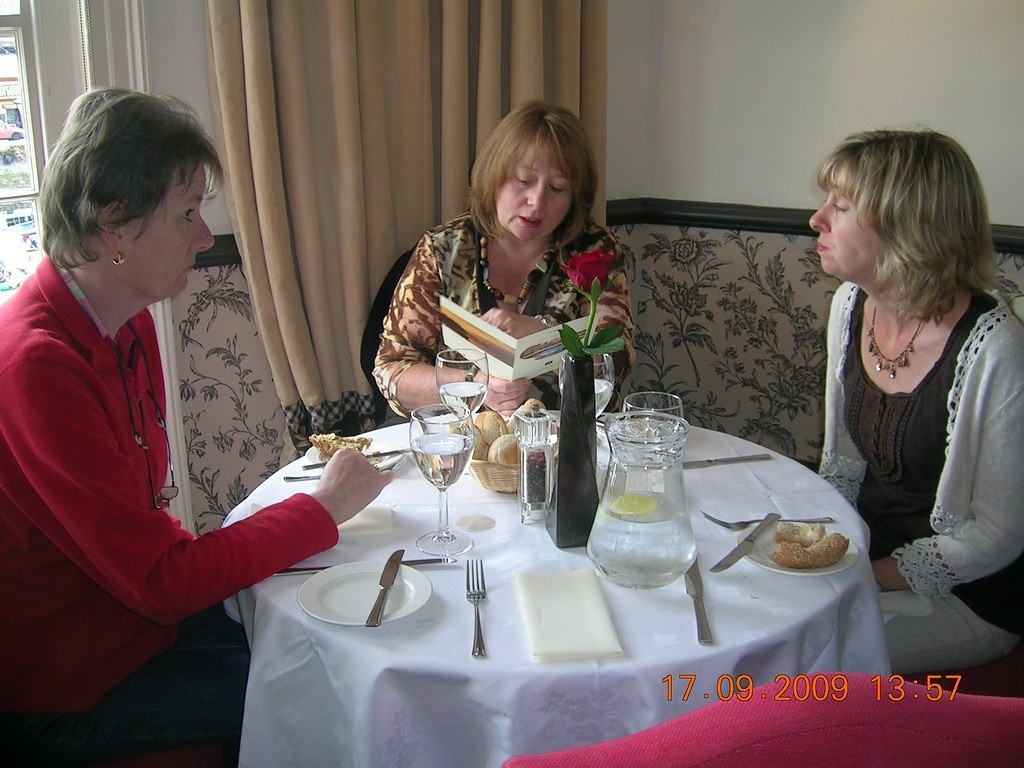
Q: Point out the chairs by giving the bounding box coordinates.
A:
[359,243,418,427]
[503,673,1024,768]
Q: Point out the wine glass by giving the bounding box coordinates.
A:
[409,403,474,556]
[435,348,489,475]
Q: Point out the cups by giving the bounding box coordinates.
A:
[558,351,615,419]
[623,392,683,421]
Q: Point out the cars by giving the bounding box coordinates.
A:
[0,119,25,141]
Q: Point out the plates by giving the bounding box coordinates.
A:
[734,524,859,577]
[298,561,433,626]
[305,436,405,470]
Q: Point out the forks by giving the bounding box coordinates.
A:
[283,457,408,482]
[467,560,487,657]
[699,510,833,531]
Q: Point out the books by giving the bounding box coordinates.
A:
[440,295,601,382]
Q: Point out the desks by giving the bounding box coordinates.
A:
[219,410,895,767]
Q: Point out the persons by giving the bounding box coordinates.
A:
[810,128,1024,674]
[0,88,395,768]
[372,100,637,429]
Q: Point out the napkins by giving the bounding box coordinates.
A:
[305,445,405,471]
[249,503,393,539]
[513,570,624,664]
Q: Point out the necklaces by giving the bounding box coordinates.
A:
[866,306,923,380]
[480,232,561,305]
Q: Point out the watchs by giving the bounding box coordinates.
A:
[534,314,556,330]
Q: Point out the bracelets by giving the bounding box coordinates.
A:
[870,560,883,593]
[461,365,486,413]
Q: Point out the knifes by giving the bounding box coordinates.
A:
[708,513,781,574]
[684,559,714,645]
[365,550,405,627]
[301,449,411,472]
[272,558,457,576]
[683,453,774,468]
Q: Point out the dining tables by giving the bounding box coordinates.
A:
[554,248,626,357]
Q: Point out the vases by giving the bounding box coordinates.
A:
[546,352,600,548]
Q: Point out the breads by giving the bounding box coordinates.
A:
[308,432,372,456]
[769,522,849,569]
[450,397,548,465]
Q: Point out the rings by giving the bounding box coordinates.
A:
[372,465,380,472]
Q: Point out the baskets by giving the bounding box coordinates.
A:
[472,454,521,495]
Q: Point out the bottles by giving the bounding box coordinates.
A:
[586,412,696,590]
[513,403,552,525]
